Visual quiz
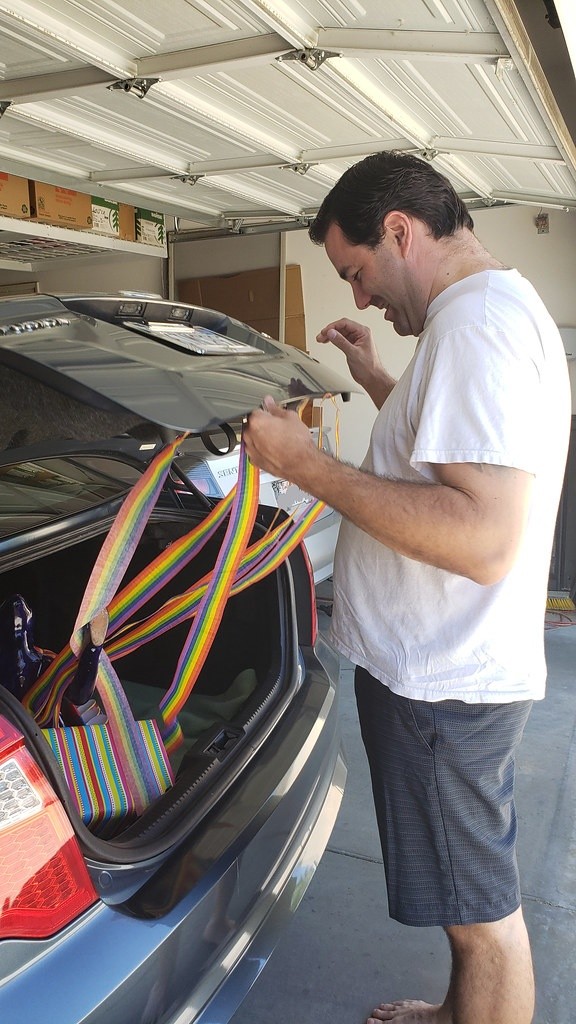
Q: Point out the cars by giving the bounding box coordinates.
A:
[170,436,342,588]
[0,289,370,1023]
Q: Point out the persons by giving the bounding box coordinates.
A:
[244,152,572,1024]
